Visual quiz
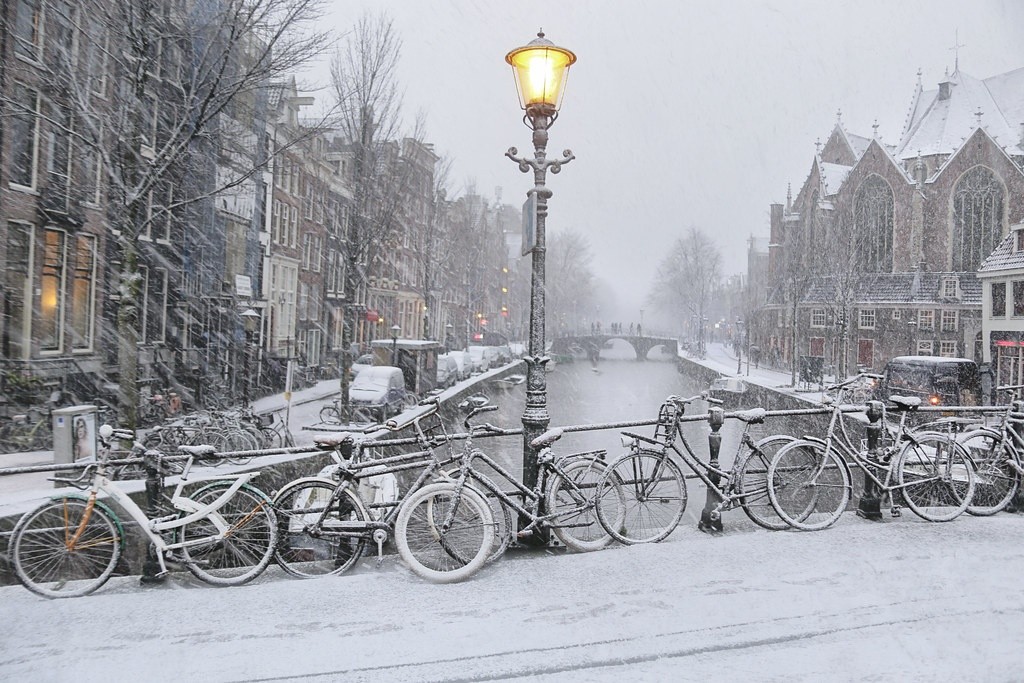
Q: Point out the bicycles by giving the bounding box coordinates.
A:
[0,359,1024,599]
[680,337,708,360]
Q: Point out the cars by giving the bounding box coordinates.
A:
[435,345,514,391]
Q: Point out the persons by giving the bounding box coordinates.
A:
[592,320,763,370]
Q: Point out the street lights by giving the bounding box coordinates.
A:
[596,305,600,330]
[702,316,709,347]
[734,318,745,374]
[445,323,453,355]
[239,307,262,408]
[502,27,570,551]
[390,322,402,365]
[907,317,916,357]
[640,306,644,334]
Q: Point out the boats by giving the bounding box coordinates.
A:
[493,372,527,390]
[459,391,491,413]
[545,360,557,372]
[709,375,748,403]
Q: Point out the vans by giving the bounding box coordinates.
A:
[350,364,407,418]
[873,356,983,431]
